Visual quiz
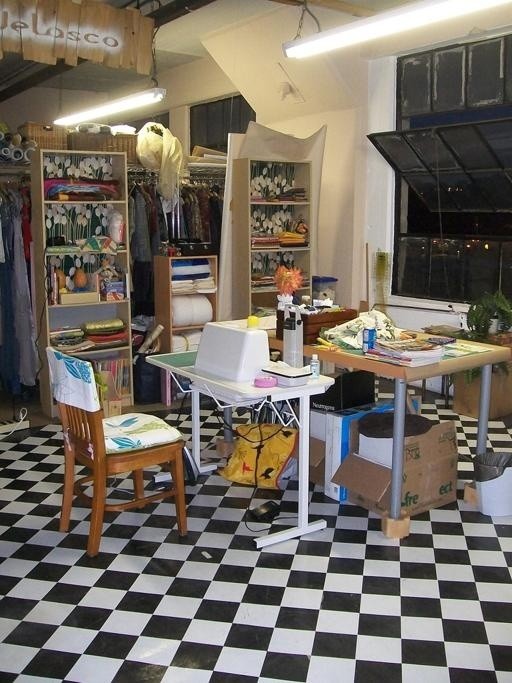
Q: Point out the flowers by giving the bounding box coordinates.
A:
[274,266,303,297]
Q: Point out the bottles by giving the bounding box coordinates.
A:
[311,353,320,382]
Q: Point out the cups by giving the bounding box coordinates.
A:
[247,314,259,327]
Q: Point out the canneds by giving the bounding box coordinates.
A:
[363,327,377,354]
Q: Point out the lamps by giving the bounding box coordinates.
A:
[282,0,510,60]
[54,26,166,125]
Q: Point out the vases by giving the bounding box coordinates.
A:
[277,293,294,310]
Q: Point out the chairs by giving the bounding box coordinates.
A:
[46,347,187,558]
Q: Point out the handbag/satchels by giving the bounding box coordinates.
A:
[218,424,298,490]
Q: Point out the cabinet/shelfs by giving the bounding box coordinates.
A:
[233,158,312,319]
[153,255,217,353]
[32,149,134,418]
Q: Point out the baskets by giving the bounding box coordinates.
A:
[17,121,138,164]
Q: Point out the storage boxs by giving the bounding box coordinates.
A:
[292,437,325,486]
[330,411,459,519]
[312,276,338,304]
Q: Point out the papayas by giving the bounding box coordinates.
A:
[54,268,66,288]
[75,268,87,288]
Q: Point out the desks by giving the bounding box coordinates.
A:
[266,326,512,538]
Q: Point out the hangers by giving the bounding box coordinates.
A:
[129,174,223,197]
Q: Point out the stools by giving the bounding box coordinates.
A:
[421,325,466,409]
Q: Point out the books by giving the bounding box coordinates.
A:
[365,337,492,368]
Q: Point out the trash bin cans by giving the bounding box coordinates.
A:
[473,452,512,515]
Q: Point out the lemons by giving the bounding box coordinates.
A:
[247,316,260,328]
